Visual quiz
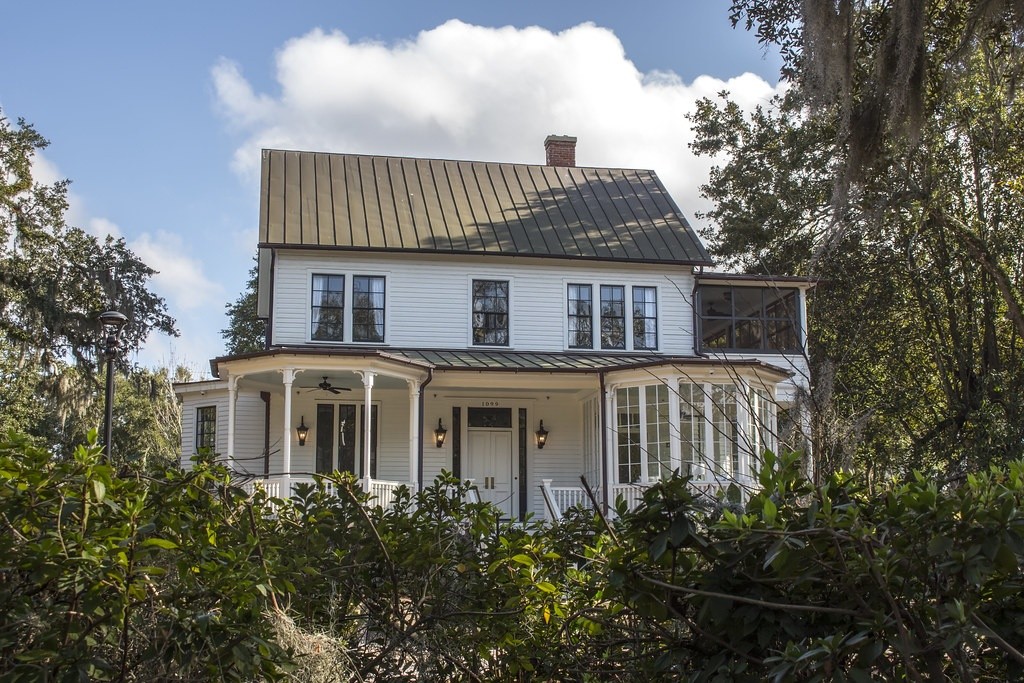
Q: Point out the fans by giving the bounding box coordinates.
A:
[703,300,733,322]
[657,385,704,420]
[298,376,352,395]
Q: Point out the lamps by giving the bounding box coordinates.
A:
[534,419,550,450]
[432,417,448,450]
[295,415,310,447]
